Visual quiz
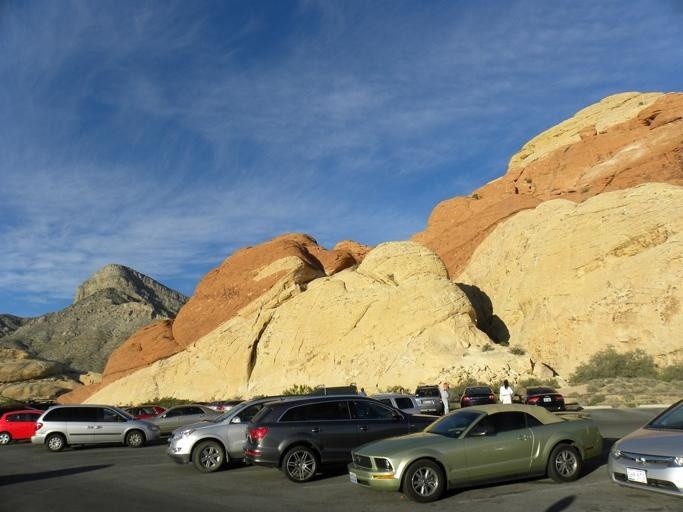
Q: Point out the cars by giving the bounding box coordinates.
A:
[608,399,682,498]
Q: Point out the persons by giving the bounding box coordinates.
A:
[499,379,513,404]
[441,388,449,414]
[359,387,366,396]
